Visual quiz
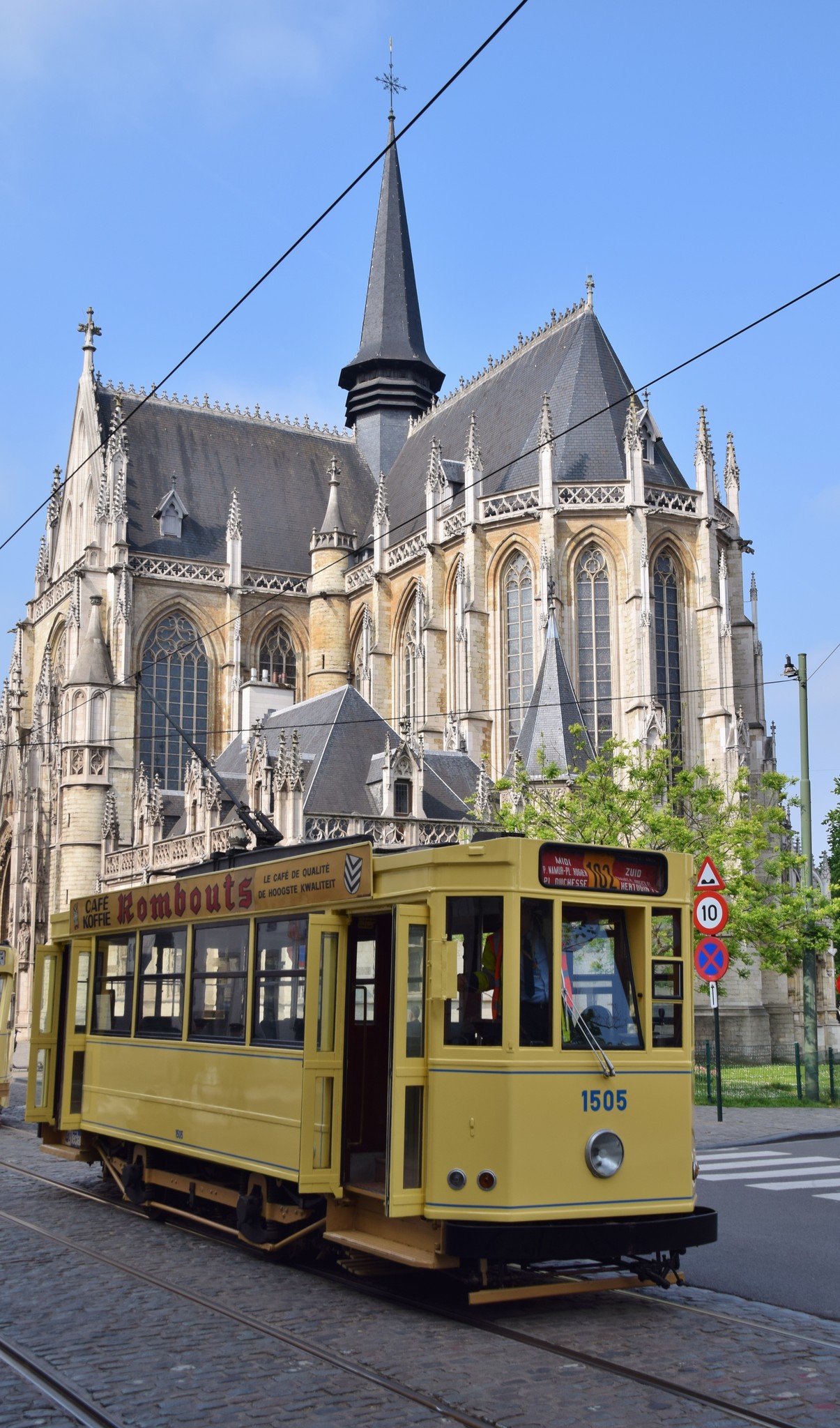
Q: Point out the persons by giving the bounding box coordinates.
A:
[455,896,600,1047]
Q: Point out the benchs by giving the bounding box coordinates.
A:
[260,1018,305,1041]
[141,1016,227,1037]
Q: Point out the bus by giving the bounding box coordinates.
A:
[24,675,717,1305]
[0,939,20,1116]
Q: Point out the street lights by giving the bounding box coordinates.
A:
[779,654,821,1103]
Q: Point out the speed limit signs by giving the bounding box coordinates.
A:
[692,891,729,935]
